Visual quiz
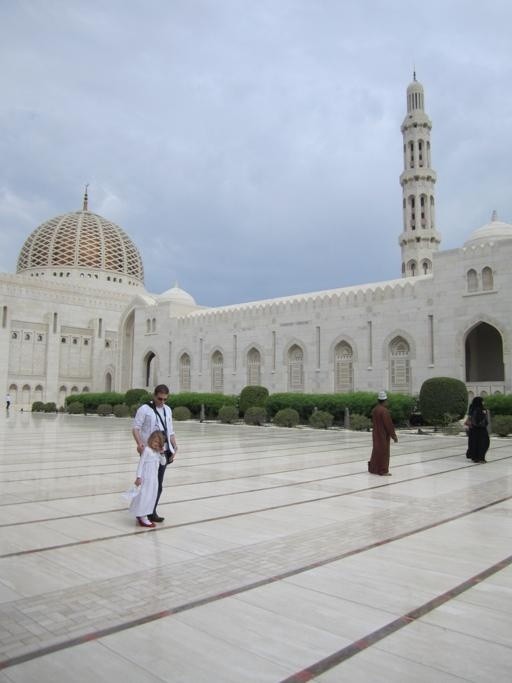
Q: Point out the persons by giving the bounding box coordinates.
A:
[131,431,164,528]
[366,391,398,476]
[6,393,13,409]
[471,397,490,463]
[130,384,177,522]
[465,404,475,460]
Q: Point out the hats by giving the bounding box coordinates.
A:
[377,391,388,401]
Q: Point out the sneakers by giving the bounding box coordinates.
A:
[147,513,165,523]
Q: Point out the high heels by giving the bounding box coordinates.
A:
[136,515,156,527]
